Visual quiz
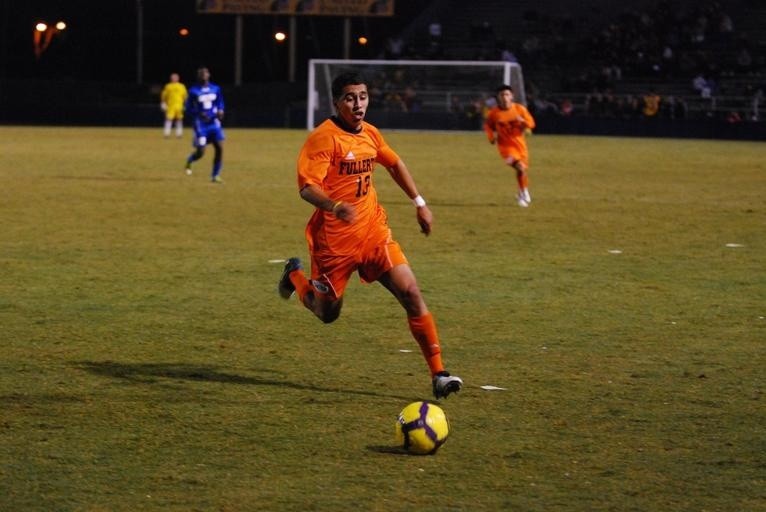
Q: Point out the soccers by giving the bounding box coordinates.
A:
[395,402,450,457]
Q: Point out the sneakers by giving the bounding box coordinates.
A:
[185,160,193,176]
[517,189,531,207]
[278,257,303,301]
[210,176,224,183]
[432,371,463,399]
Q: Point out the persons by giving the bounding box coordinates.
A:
[182,67,228,184]
[361,0,766,129]
[159,72,189,137]
[280,71,464,398]
[484,86,536,209]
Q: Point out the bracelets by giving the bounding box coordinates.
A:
[333,200,342,210]
[412,194,426,208]
